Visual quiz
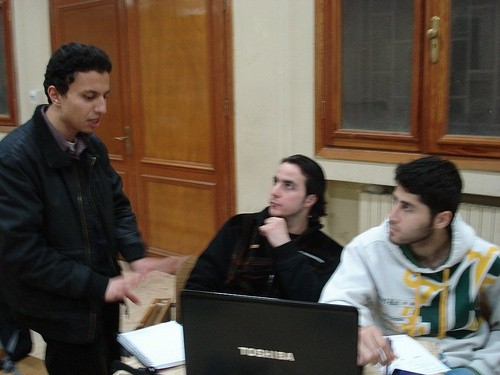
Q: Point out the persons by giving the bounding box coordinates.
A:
[318,154,499,375]
[0,41,190,374]
[182,153,344,304]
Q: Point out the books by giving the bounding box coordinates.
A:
[117,321,186,371]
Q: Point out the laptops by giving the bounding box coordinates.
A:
[180,290,358,375]
